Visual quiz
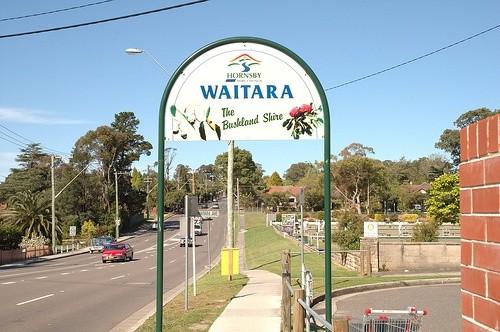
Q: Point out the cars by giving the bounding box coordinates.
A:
[194,196,219,223]
[102,242,134,263]
[152,222,158,230]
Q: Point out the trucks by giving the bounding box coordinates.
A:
[90,236,114,254]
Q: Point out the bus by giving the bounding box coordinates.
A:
[278,197,296,208]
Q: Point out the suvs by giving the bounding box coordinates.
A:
[179,237,192,247]
[194,223,202,235]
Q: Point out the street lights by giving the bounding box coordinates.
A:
[51,155,100,255]
[209,175,241,232]
[145,164,193,217]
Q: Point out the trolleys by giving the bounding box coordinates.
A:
[347,306,428,332]
